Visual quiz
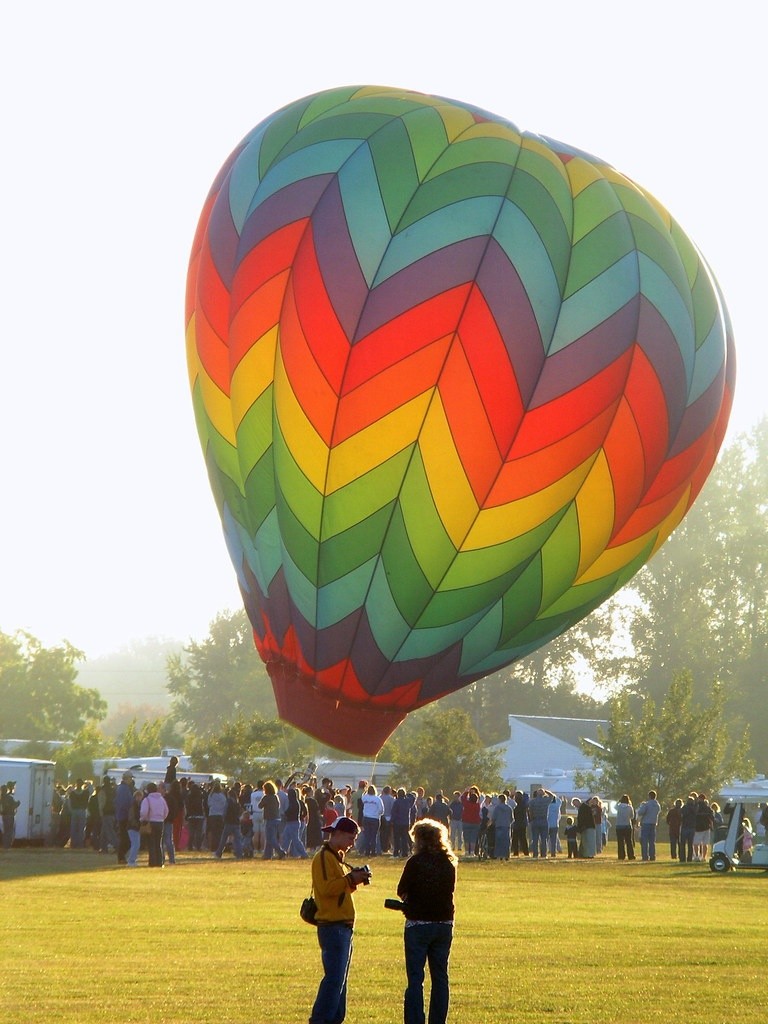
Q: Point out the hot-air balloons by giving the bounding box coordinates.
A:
[183,85,737,757]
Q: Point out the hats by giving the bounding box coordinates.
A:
[121,770,137,780]
[320,815,362,834]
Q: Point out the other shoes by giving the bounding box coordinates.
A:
[387,856,400,860]
[680,859,691,863]
[299,854,310,859]
[694,856,706,863]
[511,854,530,857]
[213,851,221,858]
[532,857,539,860]
[125,863,138,868]
[233,853,243,859]
[551,854,557,856]
[244,852,253,857]
[278,852,287,859]
[499,857,509,862]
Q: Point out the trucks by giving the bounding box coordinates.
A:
[284,761,409,793]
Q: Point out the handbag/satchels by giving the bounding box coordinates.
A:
[300,898,317,926]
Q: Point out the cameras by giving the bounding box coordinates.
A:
[384,899,408,915]
[359,864,371,885]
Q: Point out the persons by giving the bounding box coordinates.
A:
[258,782,287,860]
[127,790,143,866]
[52,757,528,860]
[528,789,554,859]
[742,818,753,853]
[0,781,21,847]
[564,796,612,859]
[754,802,768,846]
[113,771,134,864]
[361,786,384,857]
[614,790,661,861]
[397,818,459,1024]
[666,792,724,862]
[309,817,371,1024]
[547,794,563,857]
[138,783,169,867]
